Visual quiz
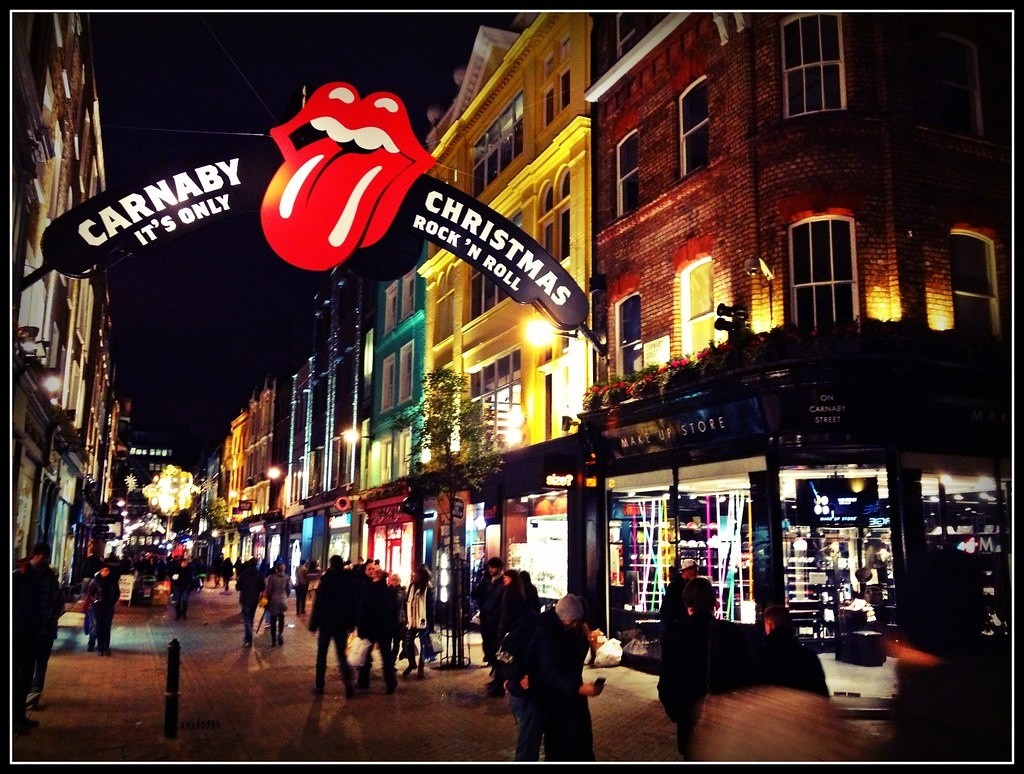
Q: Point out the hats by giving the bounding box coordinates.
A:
[679,558,698,571]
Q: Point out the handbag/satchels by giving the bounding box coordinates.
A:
[496,632,513,664]
[84,606,96,635]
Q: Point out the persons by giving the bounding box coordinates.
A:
[11,543,292,729]
[295,554,831,762]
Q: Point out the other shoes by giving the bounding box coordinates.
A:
[312,686,323,695]
[345,689,354,699]
[98,652,103,655]
[403,662,417,677]
[488,687,506,697]
[26,692,40,709]
[14,718,39,732]
[278,635,282,646]
[244,635,253,647]
[418,663,425,679]
[270,643,276,648]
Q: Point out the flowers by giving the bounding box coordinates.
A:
[585,320,902,410]
